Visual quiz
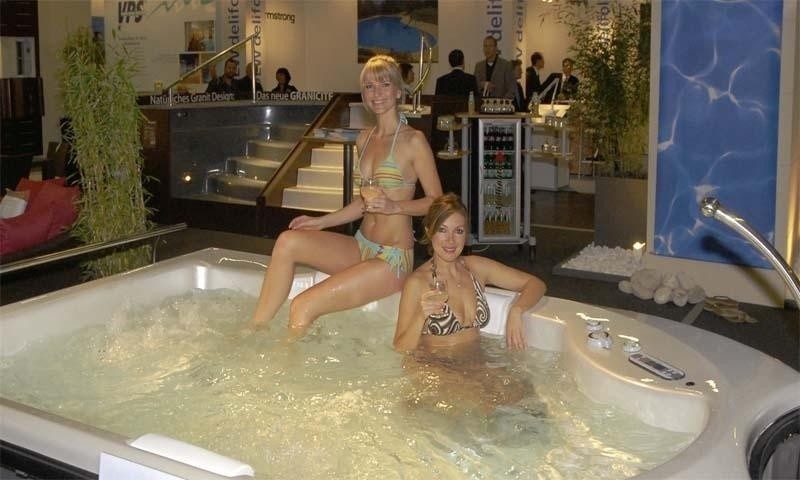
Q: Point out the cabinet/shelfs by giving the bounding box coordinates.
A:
[178,17,220,84]
[454,109,540,260]
[0,0,48,158]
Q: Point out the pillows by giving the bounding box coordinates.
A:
[1,171,84,256]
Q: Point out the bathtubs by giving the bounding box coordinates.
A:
[1,247,800,477]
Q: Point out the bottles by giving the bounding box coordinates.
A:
[530,89,539,114]
[483,124,514,151]
[484,183,512,205]
[484,209,511,235]
[468,90,475,114]
[483,154,513,180]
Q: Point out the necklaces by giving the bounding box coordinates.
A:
[447,272,468,291]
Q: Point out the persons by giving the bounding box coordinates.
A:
[542,58,579,105]
[239,63,263,100]
[511,60,526,114]
[255,54,444,340]
[431,49,479,151]
[395,193,548,414]
[269,68,299,100]
[399,63,415,98]
[526,52,544,108]
[475,38,516,107]
[205,59,241,100]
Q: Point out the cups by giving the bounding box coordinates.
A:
[154,80,163,94]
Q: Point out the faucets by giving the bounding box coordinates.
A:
[699,195,796,299]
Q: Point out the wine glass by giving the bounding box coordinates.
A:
[429,279,450,321]
[360,176,381,215]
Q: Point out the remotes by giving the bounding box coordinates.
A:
[629,353,685,380]
